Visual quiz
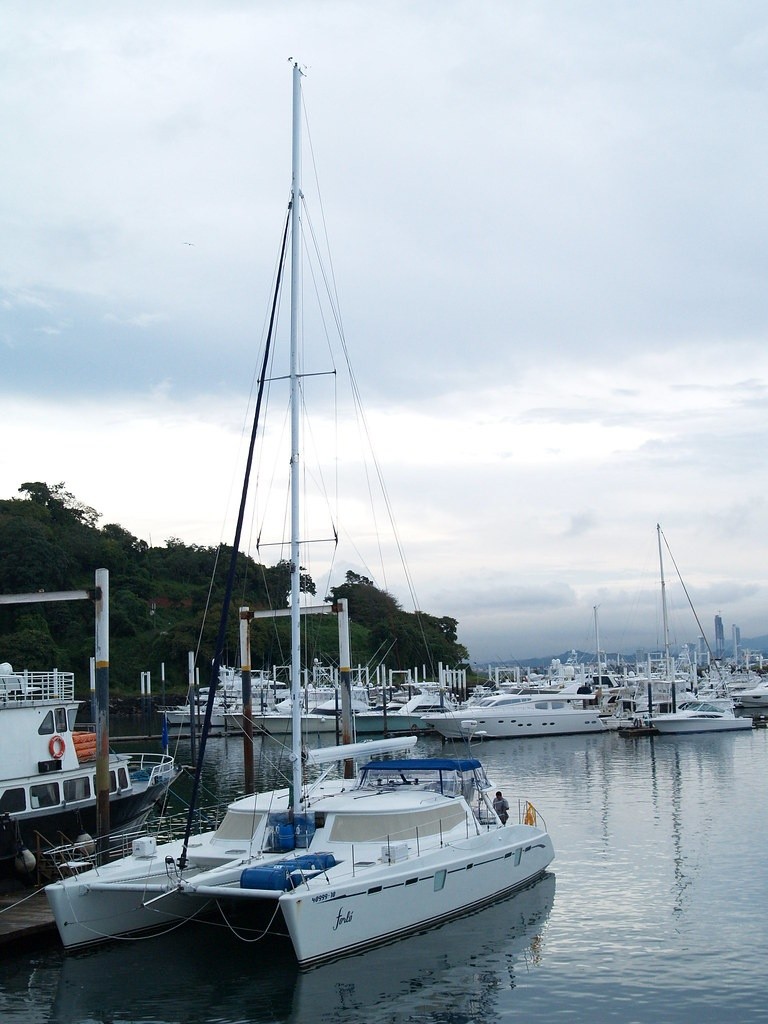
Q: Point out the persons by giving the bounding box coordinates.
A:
[493,791,509,827]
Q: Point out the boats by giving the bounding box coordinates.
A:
[0,651,173,883]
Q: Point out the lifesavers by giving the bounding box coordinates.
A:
[48,735,66,759]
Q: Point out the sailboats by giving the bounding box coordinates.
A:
[46,55,557,969]
[171,524,768,738]
